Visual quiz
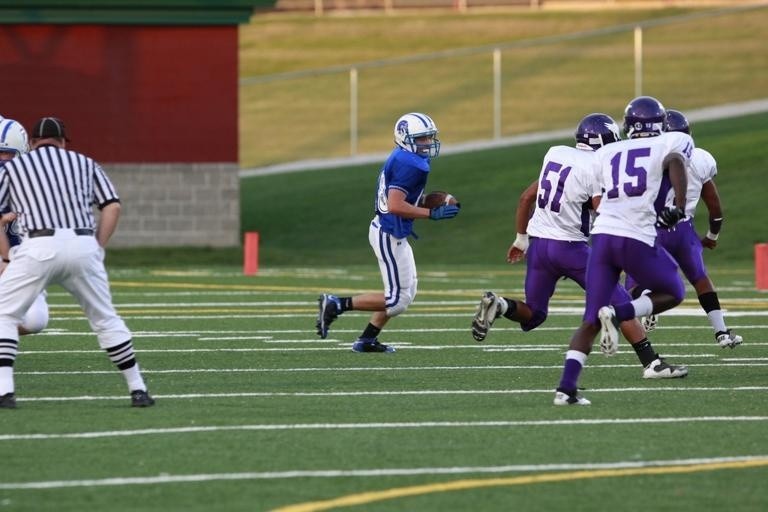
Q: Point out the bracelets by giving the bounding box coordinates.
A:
[512,232,529,254]
[705,230,720,241]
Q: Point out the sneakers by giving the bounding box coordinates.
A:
[316,293,344,339]
[716,332,744,350]
[553,390,592,406]
[0,392,17,410]
[351,335,396,354]
[472,290,498,342]
[639,289,659,333]
[595,304,619,357]
[642,358,689,380]
[130,391,155,407]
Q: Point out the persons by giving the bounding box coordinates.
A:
[620,108,743,351]
[472,113,688,379]
[0,116,154,408]
[0,118,49,337]
[553,96,687,407]
[316,112,462,354]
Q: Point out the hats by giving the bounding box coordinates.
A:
[34,117,72,142]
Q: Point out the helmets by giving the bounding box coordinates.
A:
[0,119,29,156]
[663,111,690,135]
[575,113,622,151]
[625,97,667,139]
[395,112,440,159]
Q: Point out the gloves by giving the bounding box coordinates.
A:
[652,206,687,232]
[429,200,460,221]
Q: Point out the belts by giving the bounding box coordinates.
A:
[21,227,94,239]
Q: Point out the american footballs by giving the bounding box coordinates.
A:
[422,193,457,208]
